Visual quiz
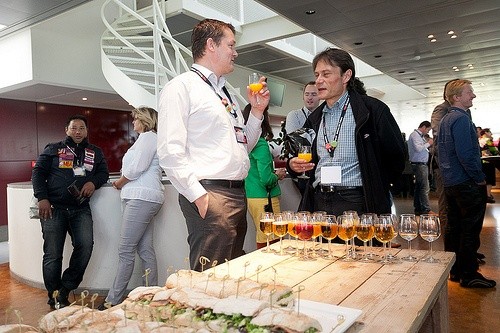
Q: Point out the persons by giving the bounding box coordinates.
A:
[437,80,497,288]
[475,127,495,203]
[31,115,109,307]
[422,134,439,191]
[407,121,440,218]
[98,107,164,311]
[286,46,405,247]
[432,79,486,281]
[156,19,271,273]
[244,103,287,248]
[285,81,321,196]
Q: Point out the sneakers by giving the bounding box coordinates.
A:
[450,271,462,281]
[460,273,496,288]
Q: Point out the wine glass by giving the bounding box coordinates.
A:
[249,73,266,108]
[259,210,442,263]
[297,145,312,178]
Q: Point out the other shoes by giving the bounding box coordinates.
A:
[48,291,72,311]
[486,197,495,203]
[477,252,485,265]
[96,301,113,312]
[426,210,439,217]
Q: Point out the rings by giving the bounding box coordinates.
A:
[302,165,305,170]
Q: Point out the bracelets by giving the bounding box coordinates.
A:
[113,182,121,190]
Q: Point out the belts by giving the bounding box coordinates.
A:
[315,186,363,194]
[200,179,245,190]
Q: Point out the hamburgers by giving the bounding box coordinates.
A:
[0,269,322,333]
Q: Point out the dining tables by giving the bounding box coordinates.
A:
[103,238,457,333]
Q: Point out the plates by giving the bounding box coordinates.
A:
[292,299,362,333]
